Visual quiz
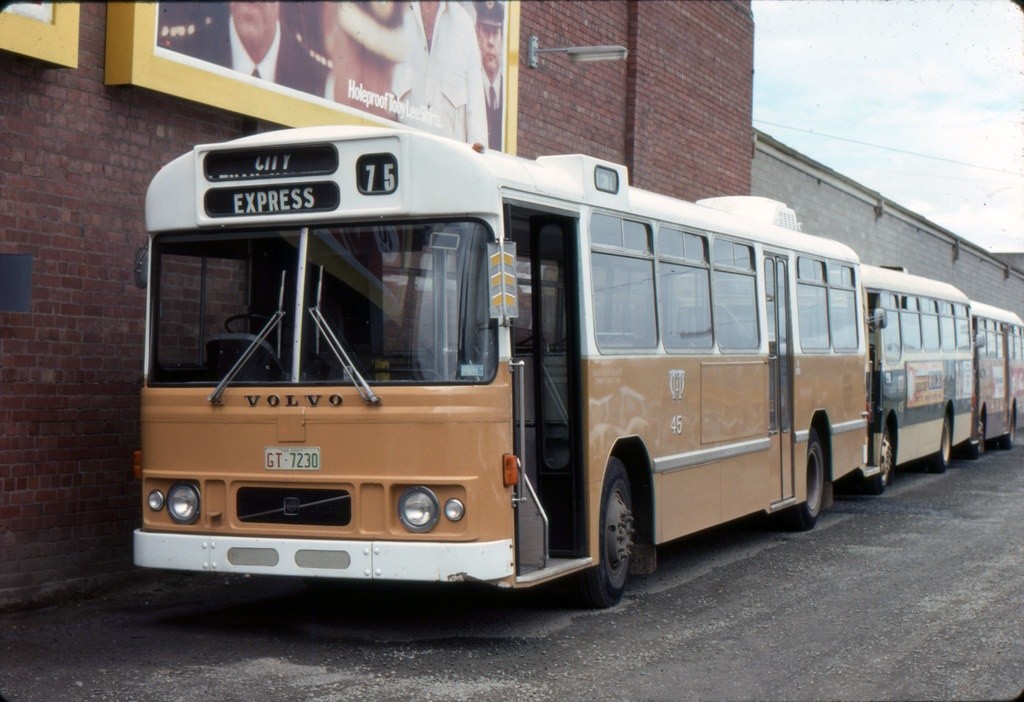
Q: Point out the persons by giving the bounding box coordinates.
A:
[170,1,324,98]
[320,1,504,153]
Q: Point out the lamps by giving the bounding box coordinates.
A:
[528,35,629,69]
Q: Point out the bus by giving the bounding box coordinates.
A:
[858,264,974,496]
[967,300,1024,459]
[134,123,881,609]
